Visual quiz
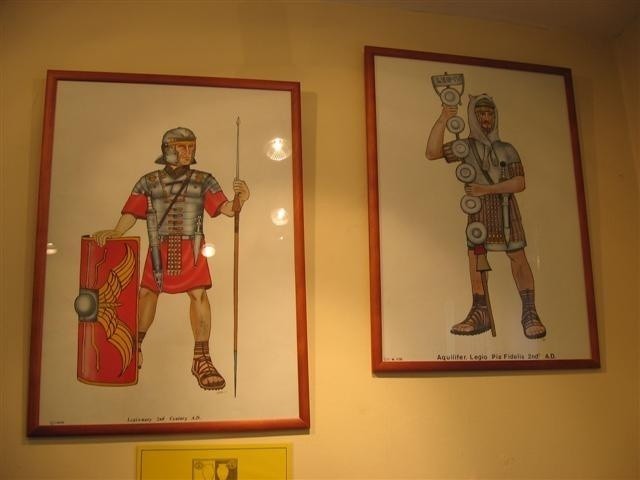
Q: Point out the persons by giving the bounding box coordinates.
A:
[425,92,548,341]
[92,126,250,391]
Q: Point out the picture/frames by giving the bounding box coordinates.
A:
[26,70,312,438]
[364,43,602,373]
